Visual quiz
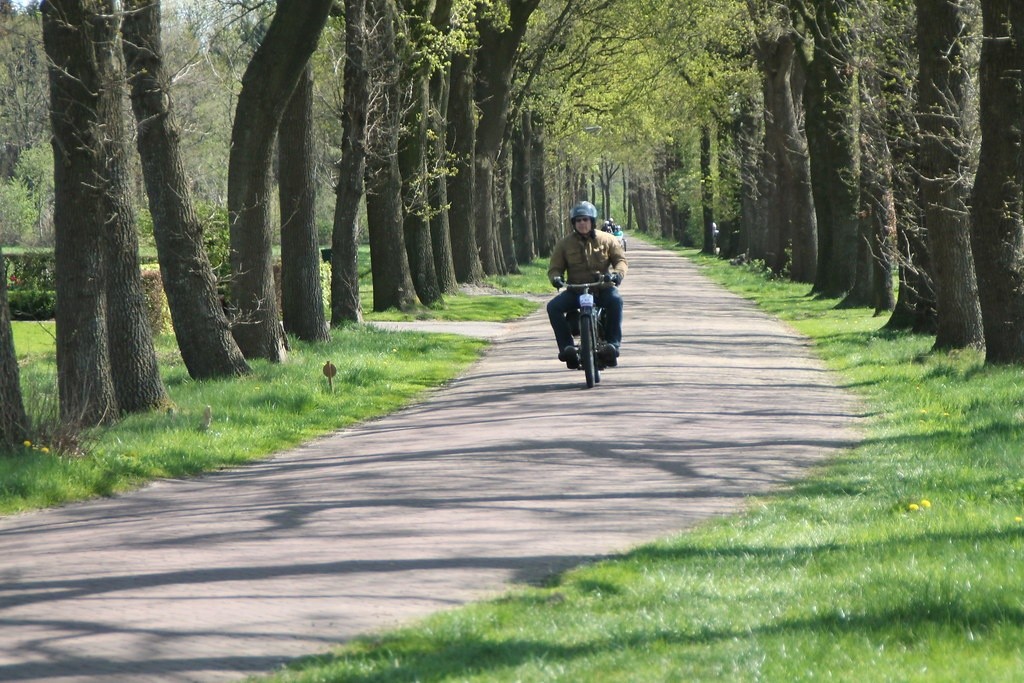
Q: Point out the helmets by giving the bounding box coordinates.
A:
[608,218,614,223]
[569,200,598,229]
[603,221,609,226]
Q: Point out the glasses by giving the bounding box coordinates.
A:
[576,217,590,222]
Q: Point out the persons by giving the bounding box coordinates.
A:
[546,200,628,368]
[601,217,623,236]
[712,221,718,251]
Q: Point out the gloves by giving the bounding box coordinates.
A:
[552,276,564,288]
[611,272,622,288]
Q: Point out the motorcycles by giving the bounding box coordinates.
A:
[602,226,626,253]
[554,273,618,387]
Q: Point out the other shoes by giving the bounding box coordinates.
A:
[603,343,621,358]
[558,343,573,362]
